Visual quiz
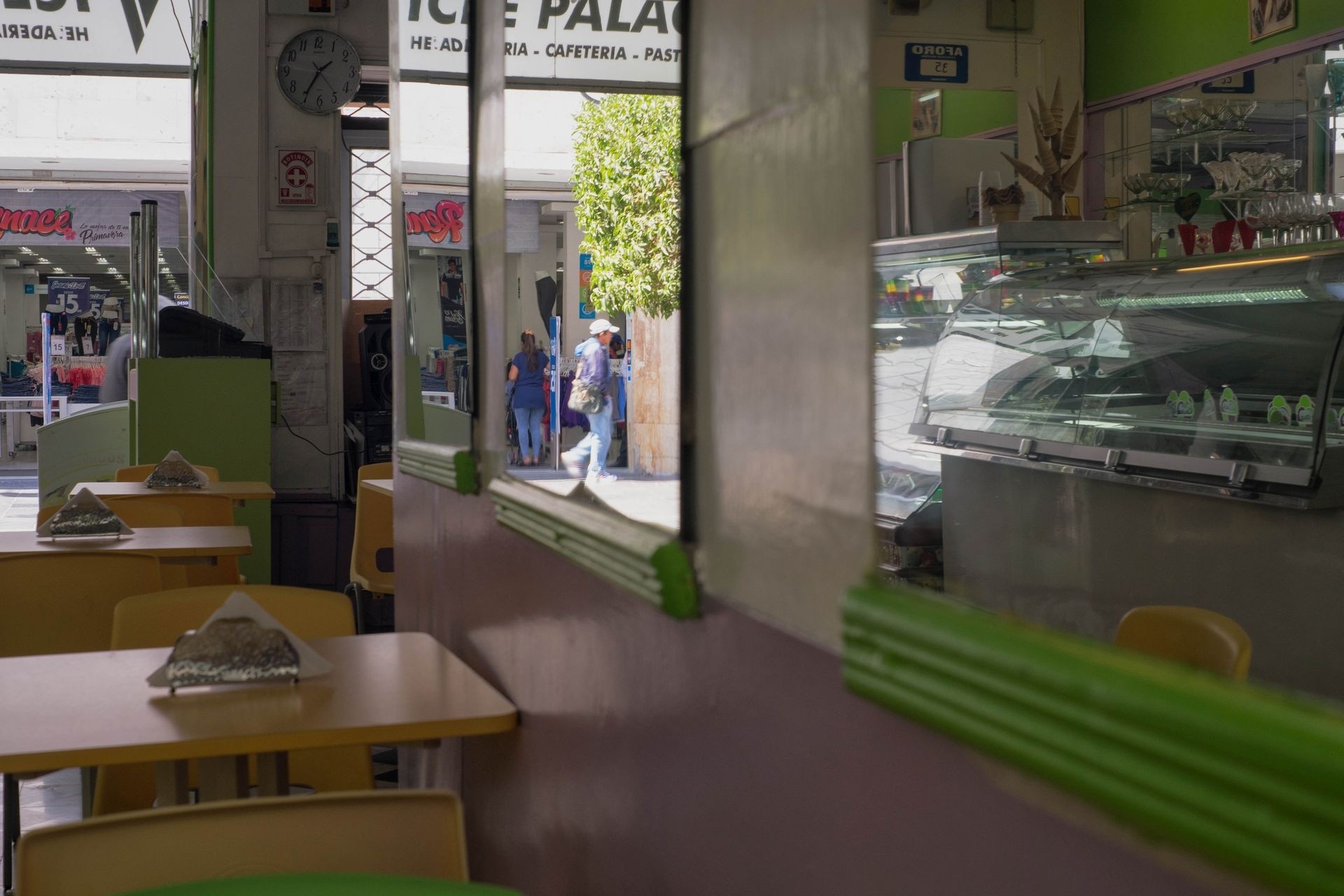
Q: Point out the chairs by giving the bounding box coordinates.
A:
[0,458,471,896]
[1108,596,1257,681]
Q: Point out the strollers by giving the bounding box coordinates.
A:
[506,387,544,466]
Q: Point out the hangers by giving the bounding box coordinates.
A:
[557,352,625,380]
[48,353,107,373]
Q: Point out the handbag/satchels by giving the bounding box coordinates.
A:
[568,379,603,415]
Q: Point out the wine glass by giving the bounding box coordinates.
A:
[1123,172,1191,203]
[1201,151,1302,201]
[1244,193,1343,249]
[1305,58,1344,112]
[1165,96,1257,138]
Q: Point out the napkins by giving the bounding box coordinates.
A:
[33,485,138,540]
[140,588,341,695]
[138,449,214,489]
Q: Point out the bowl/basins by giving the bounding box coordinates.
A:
[874,278,963,316]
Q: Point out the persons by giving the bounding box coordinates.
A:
[45,296,122,357]
[509,319,628,481]
[534,270,557,338]
[98,294,177,405]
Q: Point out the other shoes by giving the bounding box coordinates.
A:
[518,459,539,467]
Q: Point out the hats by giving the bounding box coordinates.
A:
[612,334,624,344]
[589,320,620,334]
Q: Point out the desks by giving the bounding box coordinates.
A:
[0,628,522,811]
[62,475,279,506]
[0,524,254,568]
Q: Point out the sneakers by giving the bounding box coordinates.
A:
[561,451,582,477]
[586,471,617,482]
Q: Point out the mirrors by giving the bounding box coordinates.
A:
[390,0,481,453]
[498,0,696,539]
[859,0,1344,712]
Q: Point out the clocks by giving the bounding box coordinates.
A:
[273,26,361,118]
